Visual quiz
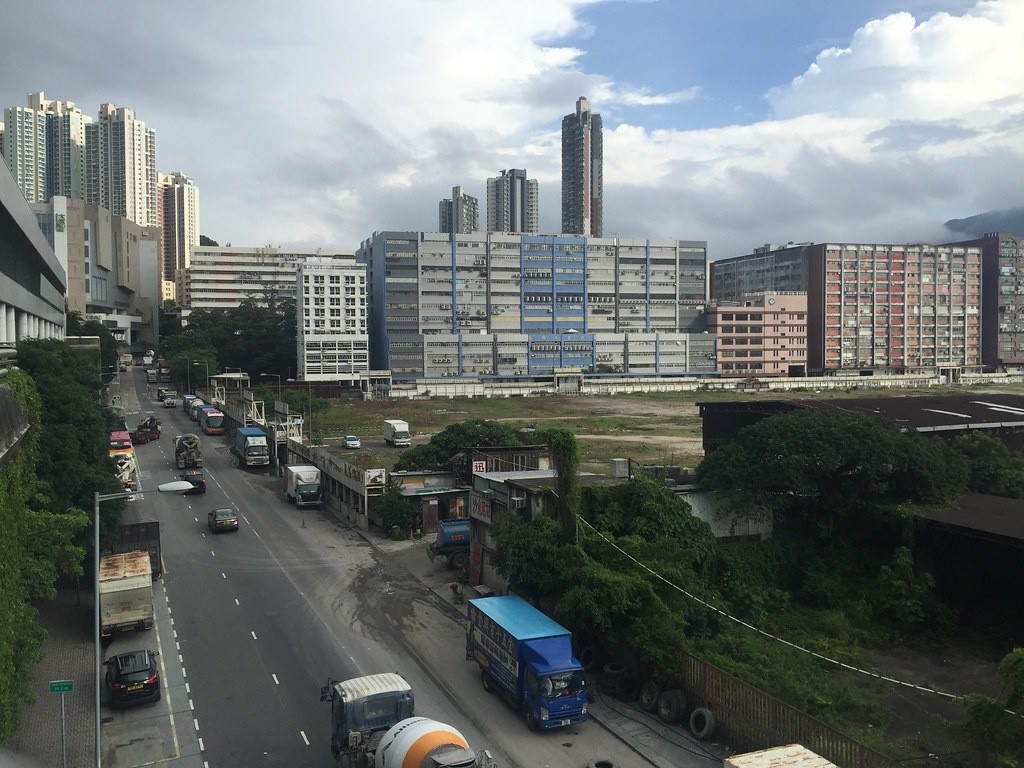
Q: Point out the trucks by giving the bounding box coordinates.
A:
[119,349,178,408]
[284,463,323,510]
[196,404,226,436]
[235,427,270,467]
[129,414,162,444]
[721,742,840,767]
[383,419,412,448]
[98,549,156,643]
[183,394,205,422]
[466,593,594,732]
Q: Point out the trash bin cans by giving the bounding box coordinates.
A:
[391,525,399,539]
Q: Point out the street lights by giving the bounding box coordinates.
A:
[259,373,281,402]
[287,378,312,448]
[224,367,242,398]
[92,480,196,768]
[178,357,209,396]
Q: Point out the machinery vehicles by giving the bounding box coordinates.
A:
[320,670,498,768]
[426,518,471,569]
[173,433,203,469]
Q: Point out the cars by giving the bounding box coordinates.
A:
[180,471,206,495]
[342,435,361,449]
[208,507,239,533]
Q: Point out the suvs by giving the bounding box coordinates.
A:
[102,651,163,707]
